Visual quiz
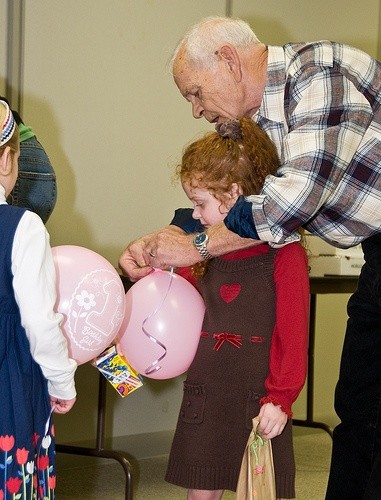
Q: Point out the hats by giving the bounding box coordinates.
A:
[0,100,17,146]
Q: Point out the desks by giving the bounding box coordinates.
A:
[55,276,359,500]
